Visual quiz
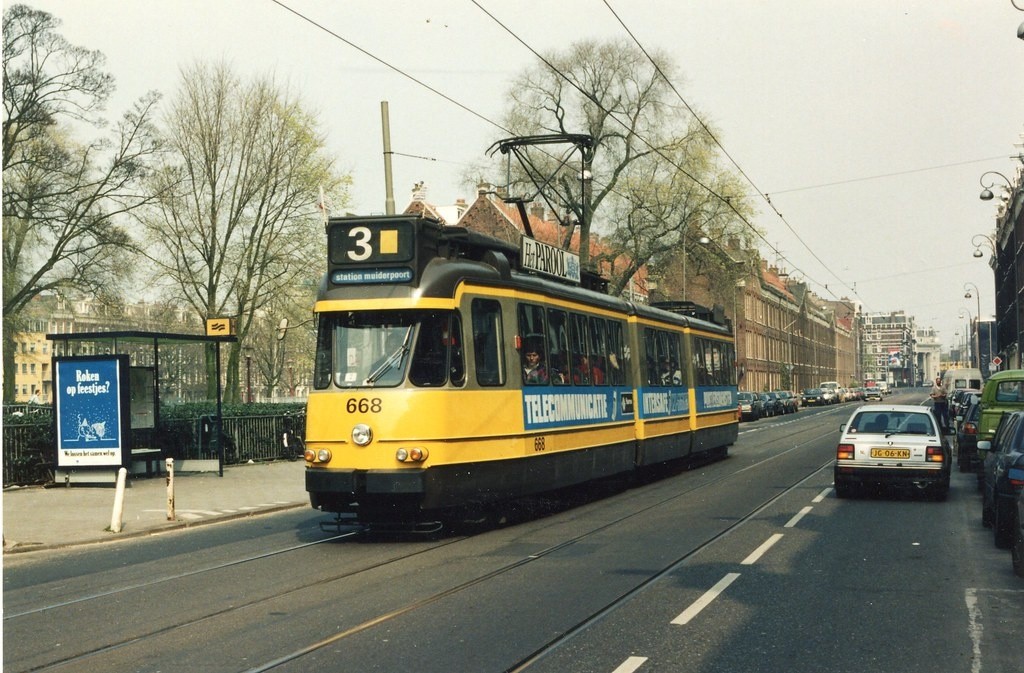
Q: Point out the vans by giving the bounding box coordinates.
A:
[876,382,888,393]
[977,370,1024,459]
[940,369,986,397]
[819,382,843,404]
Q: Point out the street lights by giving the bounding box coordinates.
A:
[958,282,980,369]
[971,234,1000,371]
[557,143,594,251]
[734,278,746,385]
[980,171,1021,370]
[243,343,254,403]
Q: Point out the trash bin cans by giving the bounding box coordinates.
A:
[200,416,219,451]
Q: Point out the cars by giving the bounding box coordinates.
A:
[801,388,825,407]
[948,388,981,472]
[922,380,933,387]
[834,405,956,500]
[841,388,885,403]
[737,390,798,422]
[977,408,1024,577]
[887,387,892,393]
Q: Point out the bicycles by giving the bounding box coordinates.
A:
[284,406,307,462]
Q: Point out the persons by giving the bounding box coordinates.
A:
[277,417,304,456]
[837,390,844,404]
[551,353,604,385]
[930,378,950,428]
[658,362,682,386]
[875,414,888,430]
[369,333,411,380]
[474,333,499,386]
[522,345,548,385]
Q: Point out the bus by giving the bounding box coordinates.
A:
[283,215,741,533]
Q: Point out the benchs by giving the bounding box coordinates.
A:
[131,448,162,479]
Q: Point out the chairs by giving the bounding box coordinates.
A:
[864,422,885,432]
[907,422,927,434]
